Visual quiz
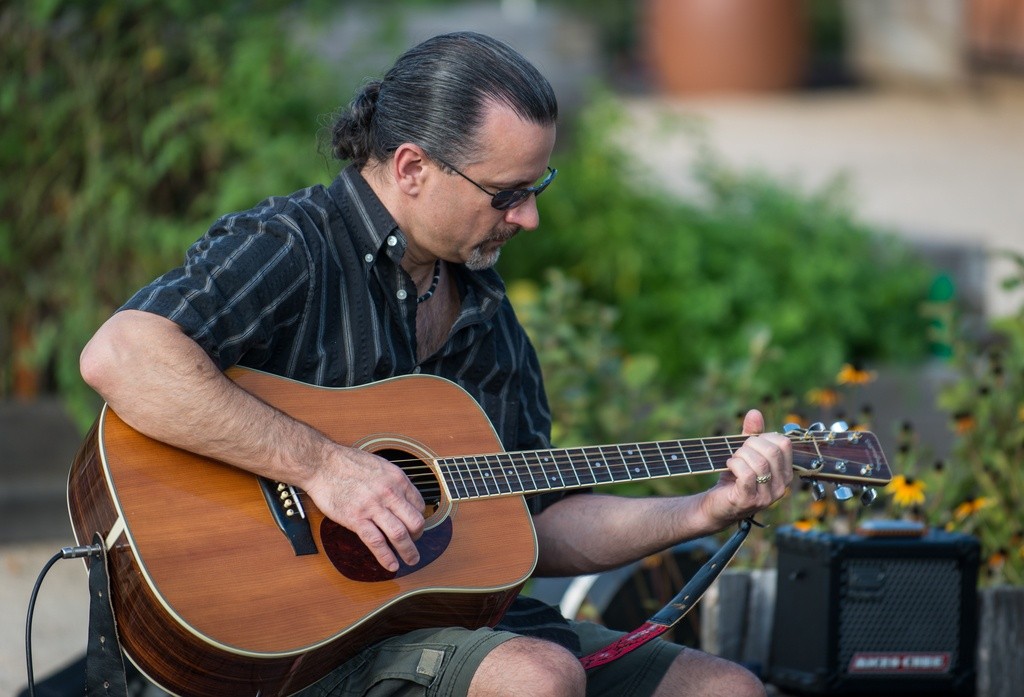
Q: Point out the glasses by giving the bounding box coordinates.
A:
[385,144,556,210]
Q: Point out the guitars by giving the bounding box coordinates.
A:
[66,362,892,697]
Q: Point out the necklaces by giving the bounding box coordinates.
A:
[415,259,441,302]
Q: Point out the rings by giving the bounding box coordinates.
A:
[756,472,772,483]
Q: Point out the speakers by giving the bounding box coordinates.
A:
[764,524,982,697]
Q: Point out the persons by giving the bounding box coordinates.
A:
[78,30,807,697]
